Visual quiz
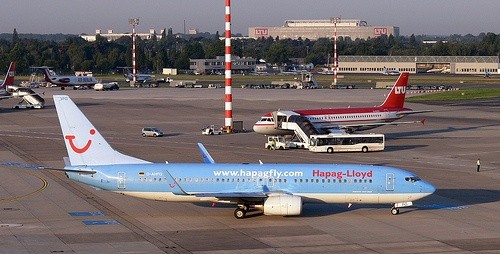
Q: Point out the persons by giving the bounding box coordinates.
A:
[476,159,480,172]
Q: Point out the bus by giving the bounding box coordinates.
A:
[309,133,384,154]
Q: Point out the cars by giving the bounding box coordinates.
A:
[201,125,222,136]
[265,136,290,151]
[141,127,164,137]
[389,71,399,75]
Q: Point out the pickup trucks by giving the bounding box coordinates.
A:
[158,77,174,82]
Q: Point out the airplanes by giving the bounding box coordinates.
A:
[30,65,98,90]
[38,94,436,218]
[0,61,17,99]
[252,71,433,135]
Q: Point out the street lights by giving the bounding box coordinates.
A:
[329,16,341,82]
[128,16,139,80]
